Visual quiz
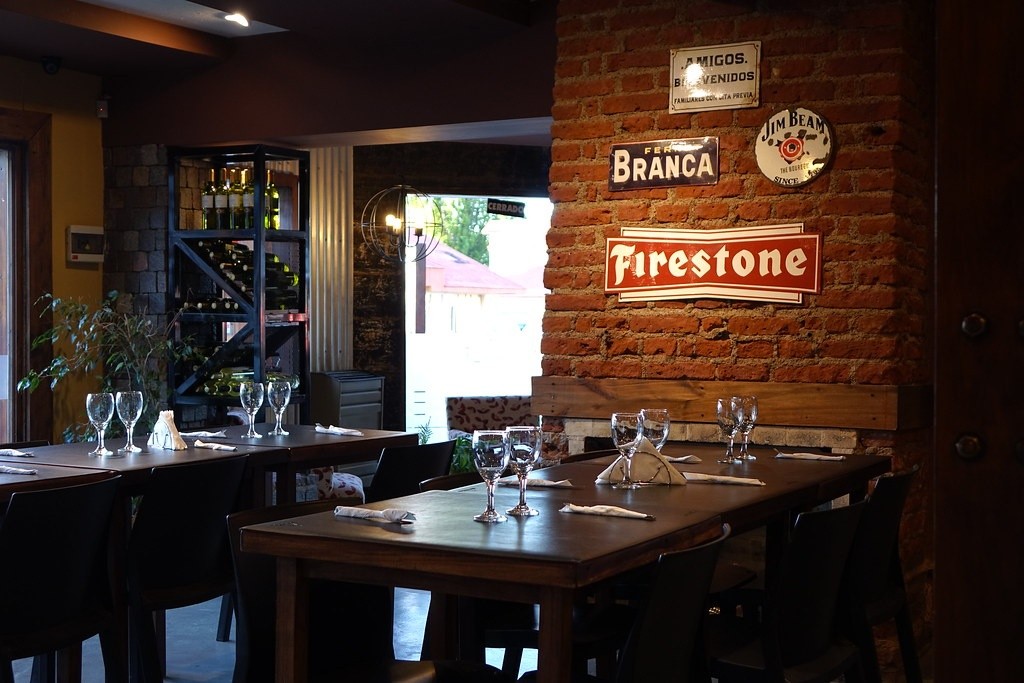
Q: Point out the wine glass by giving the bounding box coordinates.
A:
[87,393,114,457]
[116,392,143,453]
[640,409,669,452]
[611,413,644,490]
[472,430,511,523]
[717,397,744,464]
[240,382,264,438]
[732,396,758,459]
[268,382,291,436]
[506,426,542,516]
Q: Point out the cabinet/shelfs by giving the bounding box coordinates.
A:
[166,147,310,409]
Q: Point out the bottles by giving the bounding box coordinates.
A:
[241,169,255,230]
[220,250,280,284]
[265,170,280,230]
[201,169,218,230]
[177,344,247,377]
[251,290,298,306]
[238,263,298,292]
[193,367,254,396]
[265,372,300,393]
[184,298,246,314]
[199,239,249,279]
[228,168,244,230]
[215,168,230,230]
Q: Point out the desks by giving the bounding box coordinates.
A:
[239,439,891,683]
[0,425,420,683]
[0,431,132,683]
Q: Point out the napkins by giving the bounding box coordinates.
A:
[682,472,767,488]
[315,423,364,437]
[774,451,846,462]
[1,466,38,475]
[497,478,575,488]
[557,501,657,523]
[664,454,702,464]
[179,430,226,437]
[193,439,237,451]
[0,449,35,457]
[334,506,418,524]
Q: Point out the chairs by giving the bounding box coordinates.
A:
[0,437,912,683]
